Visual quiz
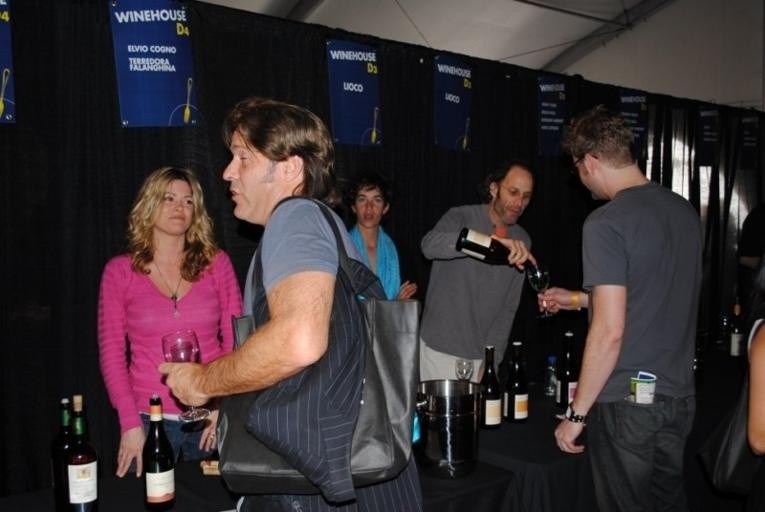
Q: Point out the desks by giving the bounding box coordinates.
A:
[0,349,748,512]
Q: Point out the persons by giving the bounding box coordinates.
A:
[729,199,765,512]
[158,97,426,512]
[419,161,539,390]
[535,105,706,512]
[344,173,418,300]
[97,162,244,480]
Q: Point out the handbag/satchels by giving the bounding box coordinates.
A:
[221,196,422,493]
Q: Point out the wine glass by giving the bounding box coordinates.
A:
[160,326,212,422]
[523,263,555,319]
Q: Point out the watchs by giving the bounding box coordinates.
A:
[564,402,588,423]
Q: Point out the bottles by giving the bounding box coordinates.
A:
[552,330,581,419]
[456,227,540,274]
[52,393,99,512]
[498,341,530,423]
[139,389,178,512]
[727,294,747,360]
[475,344,506,428]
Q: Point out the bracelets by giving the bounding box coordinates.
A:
[571,290,579,310]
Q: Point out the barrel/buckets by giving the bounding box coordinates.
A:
[417,379,482,465]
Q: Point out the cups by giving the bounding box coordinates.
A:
[453,358,473,382]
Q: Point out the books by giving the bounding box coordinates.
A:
[628,370,657,404]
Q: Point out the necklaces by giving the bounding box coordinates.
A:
[153,261,183,308]
